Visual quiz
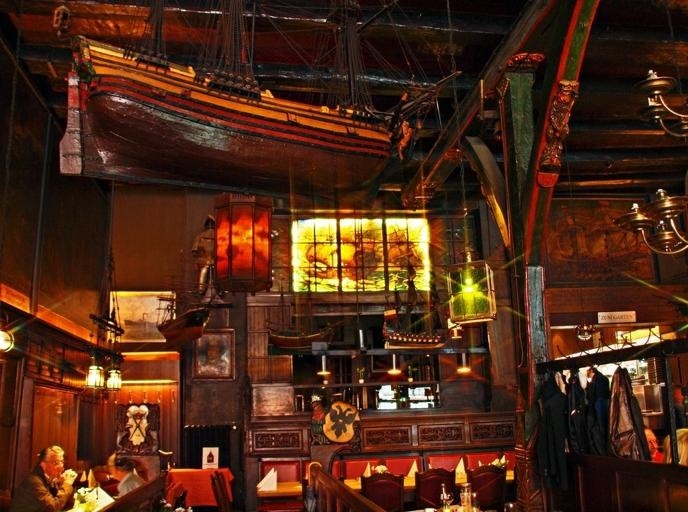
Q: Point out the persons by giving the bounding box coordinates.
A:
[107,450,147,500]
[197,341,231,377]
[6,443,80,512]
[661,428,688,465]
[643,428,664,463]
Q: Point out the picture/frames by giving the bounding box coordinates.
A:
[109,290,177,344]
[536,188,663,287]
[190,327,236,383]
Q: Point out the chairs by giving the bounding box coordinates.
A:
[257,446,517,512]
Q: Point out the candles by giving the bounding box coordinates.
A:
[461,353,467,366]
[321,355,326,374]
[359,329,364,347]
[392,354,396,370]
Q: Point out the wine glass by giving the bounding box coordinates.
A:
[443,491,455,509]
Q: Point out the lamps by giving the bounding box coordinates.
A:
[613,70,688,255]
[210,190,274,296]
[443,157,498,323]
[86,350,124,390]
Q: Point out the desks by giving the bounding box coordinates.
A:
[167,467,235,508]
